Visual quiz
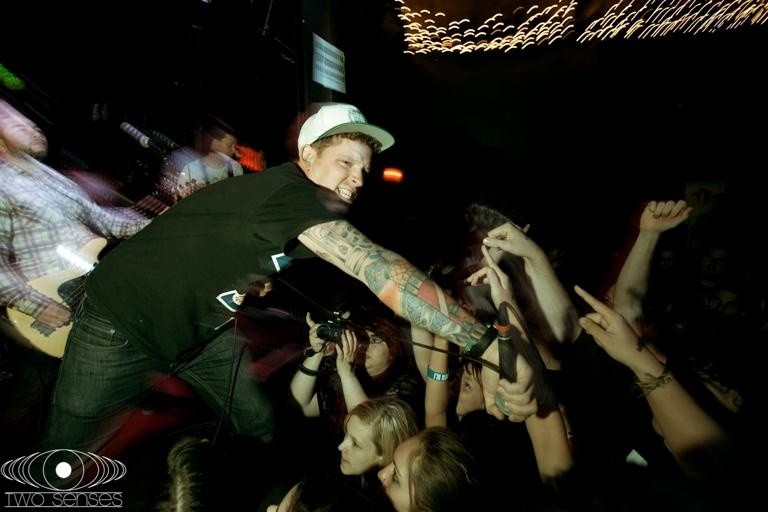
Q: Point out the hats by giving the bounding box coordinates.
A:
[297,101,395,156]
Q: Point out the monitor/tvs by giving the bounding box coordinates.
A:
[308,27,349,98]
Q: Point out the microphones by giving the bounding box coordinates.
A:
[151,129,181,150]
[495,304,516,417]
[120,121,162,152]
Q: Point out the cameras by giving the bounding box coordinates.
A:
[317,302,369,354]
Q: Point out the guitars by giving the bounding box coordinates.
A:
[5,177,208,361]
